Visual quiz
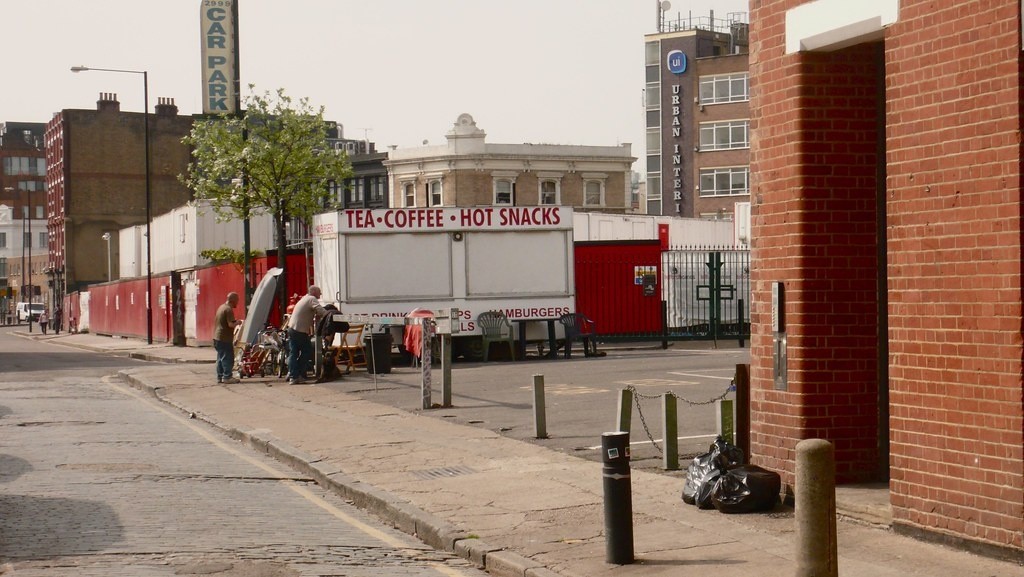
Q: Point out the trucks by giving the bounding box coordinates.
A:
[17,302,46,322]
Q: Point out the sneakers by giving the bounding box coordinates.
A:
[218,376,240,383]
[289,375,305,384]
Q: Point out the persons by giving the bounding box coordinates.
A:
[39,310,49,335]
[286,285,330,384]
[213,292,242,384]
[53,304,62,334]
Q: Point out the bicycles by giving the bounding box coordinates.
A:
[232,323,316,379]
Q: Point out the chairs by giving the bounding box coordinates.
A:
[335,324,367,373]
[560,313,596,358]
[477,311,516,364]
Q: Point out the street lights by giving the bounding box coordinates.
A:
[69,66,154,345]
[102,232,112,282]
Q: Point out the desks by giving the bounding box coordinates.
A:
[512,318,562,361]
